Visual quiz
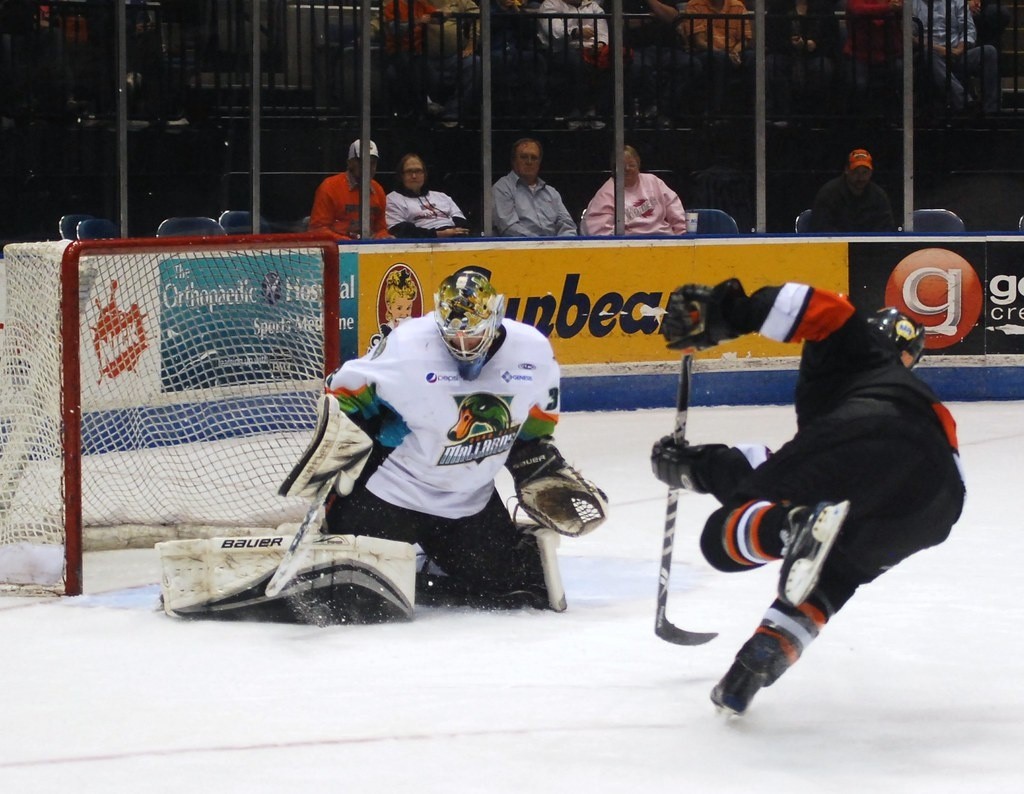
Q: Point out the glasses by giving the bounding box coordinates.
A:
[405,168,422,174]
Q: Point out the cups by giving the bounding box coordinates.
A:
[686,213,697,232]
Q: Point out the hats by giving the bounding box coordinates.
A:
[848,149,874,173]
[349,139,379,161]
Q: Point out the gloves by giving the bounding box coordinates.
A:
[660,277,747,354]
[651,432,730,494]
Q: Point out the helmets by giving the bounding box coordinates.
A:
[867,306,925,371]
[436,269,498,361]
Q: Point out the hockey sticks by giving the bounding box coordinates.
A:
[263,470,340,597]
[650,354,720,646]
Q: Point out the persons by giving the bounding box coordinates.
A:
[385,153,477,238]
[809,148,898,232]
[649,279,967,714]
[268,267,614,625]
[307,139,396,240]
[579,145,689,237]
[349,0,1016,129]
[491,137,577,238]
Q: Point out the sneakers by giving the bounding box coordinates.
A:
[710,659,766,731]
[777,501,852,608]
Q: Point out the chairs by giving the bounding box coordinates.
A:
[75,218,121,241]
[219,211,273,235]
[156,217,226,238]
[795,209,812,234]
[326,14,358,48]
[914,208,966,232]
[58,214,95,240]
[686,208,740,234]
